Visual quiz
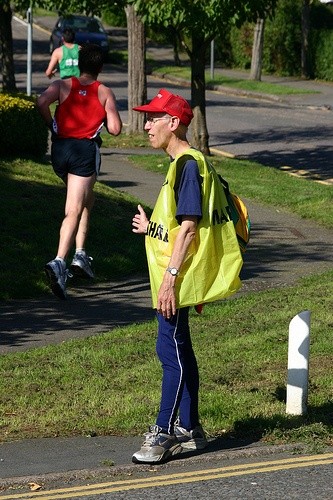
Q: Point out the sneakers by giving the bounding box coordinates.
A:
[70,253,95,280]
[45,258,73,301]
[173,416,208,450]
[131,424,183,465]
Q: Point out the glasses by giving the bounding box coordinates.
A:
[147,115,169,123]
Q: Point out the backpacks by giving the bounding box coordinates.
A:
[217,173,251,258]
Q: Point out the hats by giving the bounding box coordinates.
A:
[132,87,194,127]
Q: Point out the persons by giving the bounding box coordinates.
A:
[46,27,81,79]
[39,44,123,299]
[132,87,222,464]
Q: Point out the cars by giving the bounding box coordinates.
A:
[49,14,110,55]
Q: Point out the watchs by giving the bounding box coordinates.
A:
[167,268,179,276]
